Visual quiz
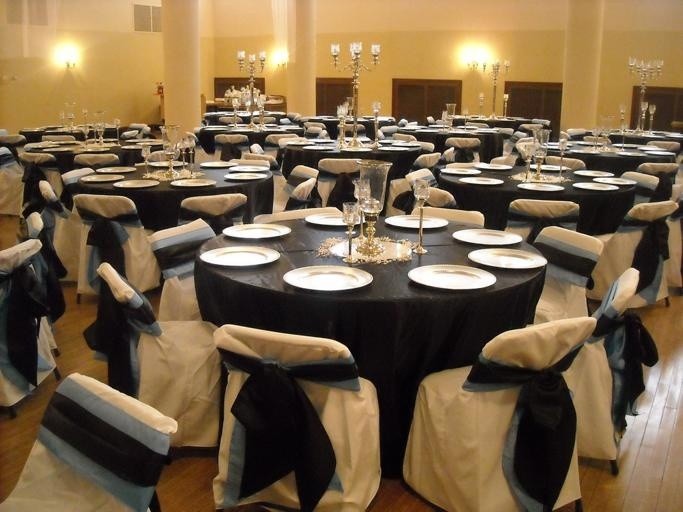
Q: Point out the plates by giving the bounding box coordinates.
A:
[81,139,271,191]
[635,146,676,156]
[639,130,683,140]
[21,109,167,153]
[442,125,646,200]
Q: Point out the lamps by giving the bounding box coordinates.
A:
[628,56,664,133]
[331,42,380,148]
[237,51,266,129]
[483,58,510,120]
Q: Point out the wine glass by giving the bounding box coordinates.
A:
[341,202,359,264]
[617,102,656,138]
[356,159,394,255]
[411,178,431,254]
[352,179,371,243]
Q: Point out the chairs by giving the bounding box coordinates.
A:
[0,111,683,512]
